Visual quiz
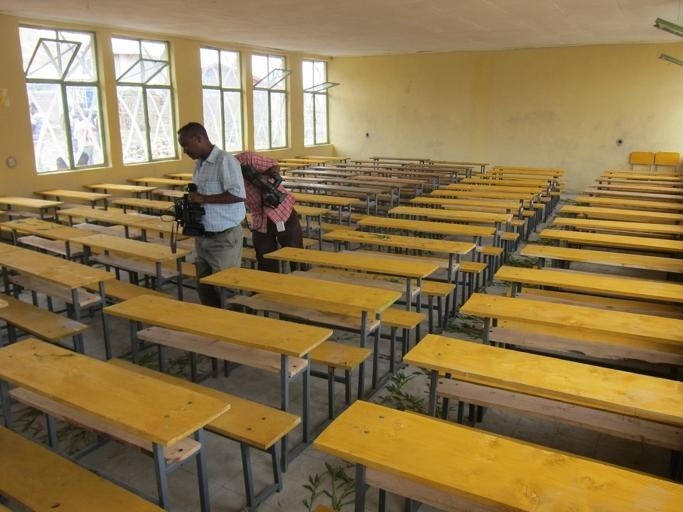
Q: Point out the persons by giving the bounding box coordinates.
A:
[234,150,307,275]
[177,122,248,314]
